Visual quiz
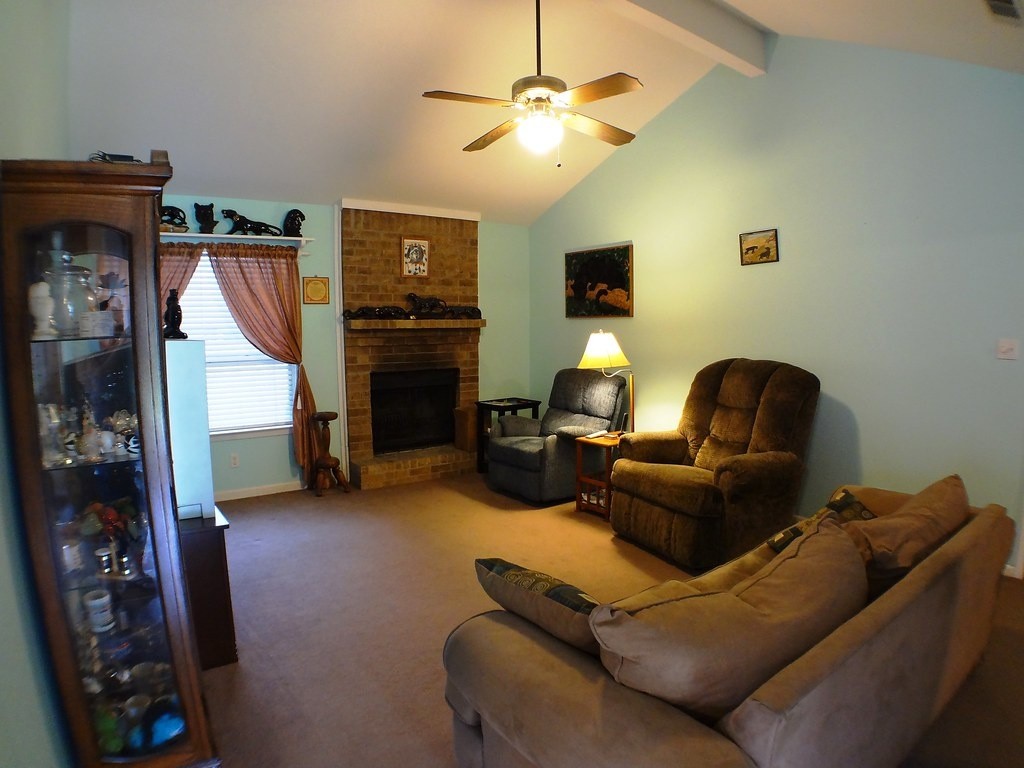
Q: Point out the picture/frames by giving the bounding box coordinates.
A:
[401,236,430,279]
[739,228,779,265]
[303,276,330,305]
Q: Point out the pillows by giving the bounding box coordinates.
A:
[472,553,598,658]
[762,489,880,555]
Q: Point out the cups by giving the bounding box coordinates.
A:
[57,539,82,574]
[84,590,115,633]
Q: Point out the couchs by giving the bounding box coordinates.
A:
[483,369,626,507]
[609,357,820,577]
[443,474,1017,768]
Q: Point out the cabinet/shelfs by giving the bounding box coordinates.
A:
[0,148,240,768]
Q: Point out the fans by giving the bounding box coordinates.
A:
[421,0,644,152]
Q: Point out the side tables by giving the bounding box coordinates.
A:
[476,397,543,450]
[575,431,629,521]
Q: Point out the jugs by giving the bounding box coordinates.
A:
[42,251,100,334]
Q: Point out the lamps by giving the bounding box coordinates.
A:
[516,116,564,168]
[577,328,635,437]
